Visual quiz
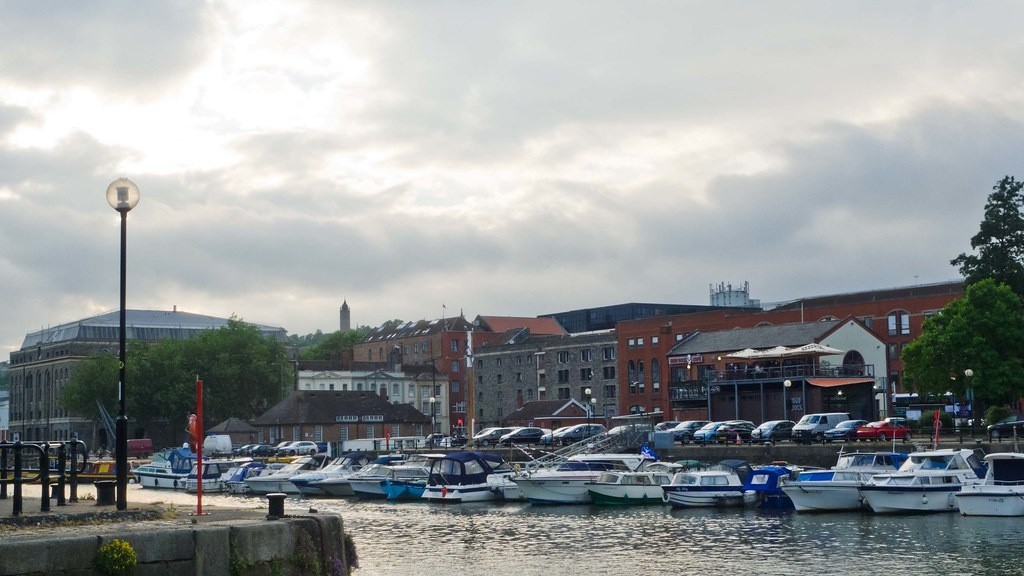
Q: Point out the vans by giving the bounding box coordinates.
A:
[127,438,153,460]
[791,413,852,443]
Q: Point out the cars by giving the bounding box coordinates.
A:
[750,419,797,444]
[656,420,682,431]
[856,421,912,442]
[693,421,726,444]
[540,425,574,445]
[716,421,758,444]
[824,419,873,444]
[498,425,546,447]
[986,414,1024,438]
[666,420,713,445]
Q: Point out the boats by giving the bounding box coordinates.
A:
[6,423,1024,517]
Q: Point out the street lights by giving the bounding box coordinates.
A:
[585,387,596,437]
[106,177,141,511]
[429,396,436,444]
[783,379,792,440]
[965,369,976,436]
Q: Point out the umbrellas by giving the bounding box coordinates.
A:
[722,343,845,371]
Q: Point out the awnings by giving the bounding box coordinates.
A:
[806,378,875,387]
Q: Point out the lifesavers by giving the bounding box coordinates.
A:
[188,414,199,454]
[934,420,942,429]
[514,464,521,474]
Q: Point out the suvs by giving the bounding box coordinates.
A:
[473,428,515,448]
[548,423,607,447]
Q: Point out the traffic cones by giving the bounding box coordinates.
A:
[735,433,742,445]
